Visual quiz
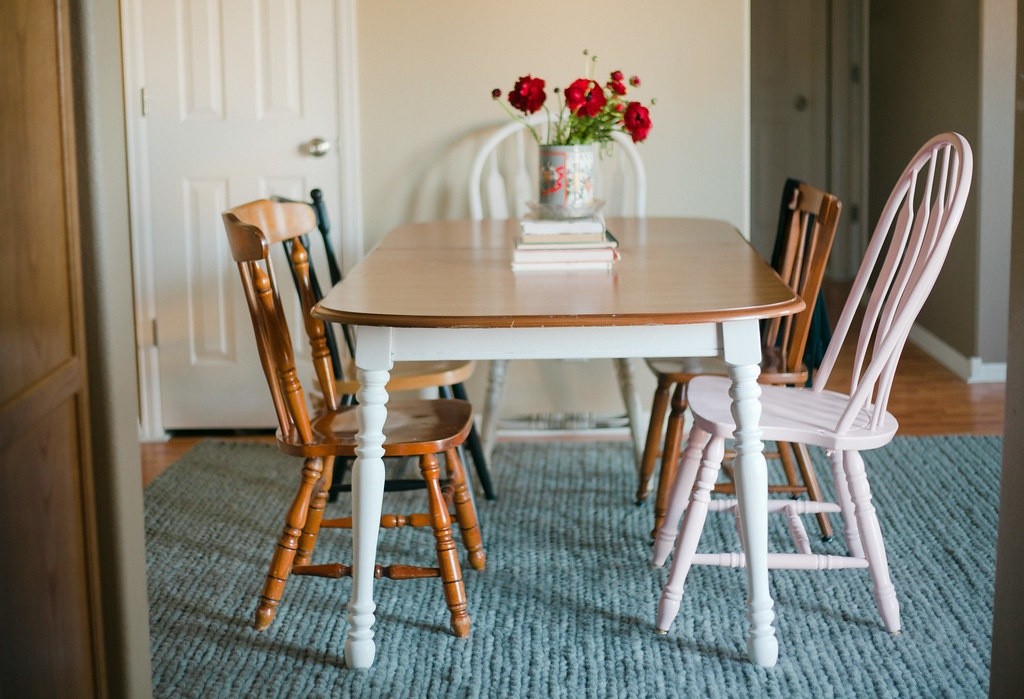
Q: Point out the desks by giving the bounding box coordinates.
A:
[309,216,807,670]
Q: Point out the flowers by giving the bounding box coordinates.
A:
[490,50,658,161]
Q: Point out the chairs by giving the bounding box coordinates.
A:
[271,189,498,501]
[634,175,842,550]
[220,197,487,640]
[651,130,972,634]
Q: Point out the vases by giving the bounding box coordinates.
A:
[537,143,598,211]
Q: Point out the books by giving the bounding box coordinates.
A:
[510,212,622,272]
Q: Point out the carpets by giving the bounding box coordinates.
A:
[142,431,1003,699]
